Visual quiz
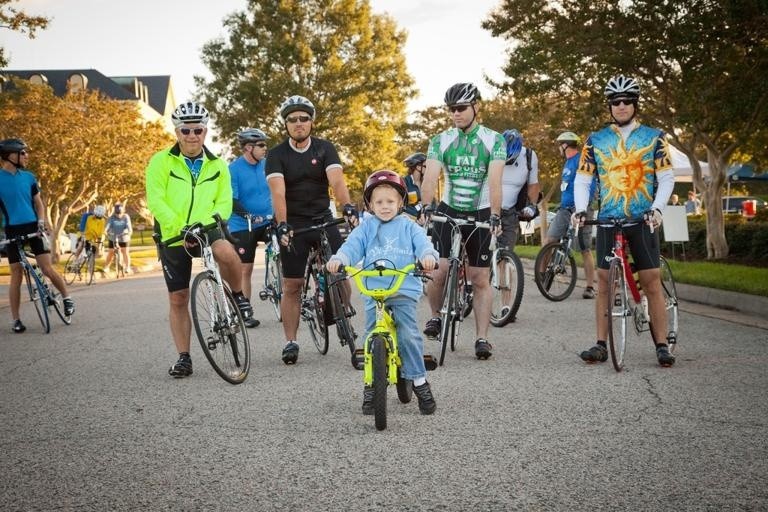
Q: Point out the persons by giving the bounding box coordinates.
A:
[538,131,595,299]
[104,205,132,273]
[402,153,427,217]
[501,129,541,324]
[75,205,107,284]
[228,128,272,328]
[668,194,682,205]
[144,101,260,377]
[0,138,75,334]
[570,73,676,367]
[415,83,507,360]
[686,191,701,213]
[265,94,359,365]
[326,170,440,414]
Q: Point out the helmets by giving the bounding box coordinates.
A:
[444,82,481,106]
[0,139,27,158]
[171,103,211,126]
[363,169,409,212]
[115,205,123,213]
[239,128,265,146]
[404,153,427,167]
[604,75,640,99]
[94,206,105,217]
[558,132,581,142]
[280,95,316,117]
[503,130,522,166]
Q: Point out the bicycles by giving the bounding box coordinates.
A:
[254,215,287,323]
[534,206,591,302]
[152,212,257,387]
[328,254,445,427]
[63,233,97,287]
[456,205,537,329]
[0,226,74,335]
[578,206,681,374]
[105,230,132,279]
[419,205,505,362]
[288,211,360,371]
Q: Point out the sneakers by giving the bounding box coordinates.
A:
[424,318,440,336]
[282,343,299,363]
[169,356,191,377]
[582,284,594,299]
[12,319,26,333]
[501,308,516,323]
[654,342,675,366]
[362,385,376,414]
[580,344,608,362]
[413,383,435,414]
[475,338,492,360]
[62,296,75,316]
[236,292,259,327]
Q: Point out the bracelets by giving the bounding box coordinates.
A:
[37,219,46,224]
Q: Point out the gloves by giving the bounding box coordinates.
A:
[37,223,49,236]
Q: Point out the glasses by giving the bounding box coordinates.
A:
[450,105,470,113]
[609,100,634,106]
[286,116,312,123]
[251,143,266,147]
[179,127,203,136]
